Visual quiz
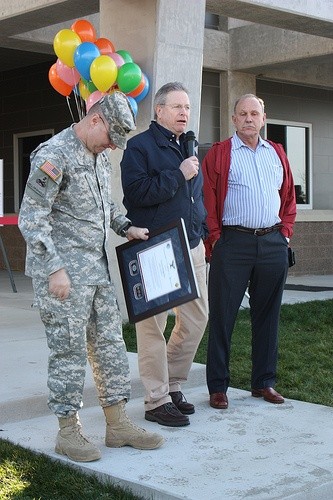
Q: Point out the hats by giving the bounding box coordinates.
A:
[99,91,136,151]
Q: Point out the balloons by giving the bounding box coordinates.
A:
[74,43,101,81]
[55,59,79,86]
[53,30,81,68]
[49,64,74,96]
[79,39,149,115]
[71,20,96,45]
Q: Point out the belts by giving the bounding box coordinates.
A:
[225,223,284,236]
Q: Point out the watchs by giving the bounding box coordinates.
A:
[121,223,132,237]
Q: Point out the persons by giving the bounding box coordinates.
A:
[121,83,209,427]
[18,91,163,461]
[201,94,296,409]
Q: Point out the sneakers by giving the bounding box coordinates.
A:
[145,402,190,426]
[169,391,195,413]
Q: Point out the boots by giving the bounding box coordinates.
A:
[104,399,165,450]
[55,412,101,461]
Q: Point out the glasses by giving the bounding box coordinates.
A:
[160,103,191,112]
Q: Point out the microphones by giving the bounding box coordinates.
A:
[185,131,195,157]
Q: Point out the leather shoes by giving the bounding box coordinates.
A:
[252,386,284,403]
[210,392,228,408]
